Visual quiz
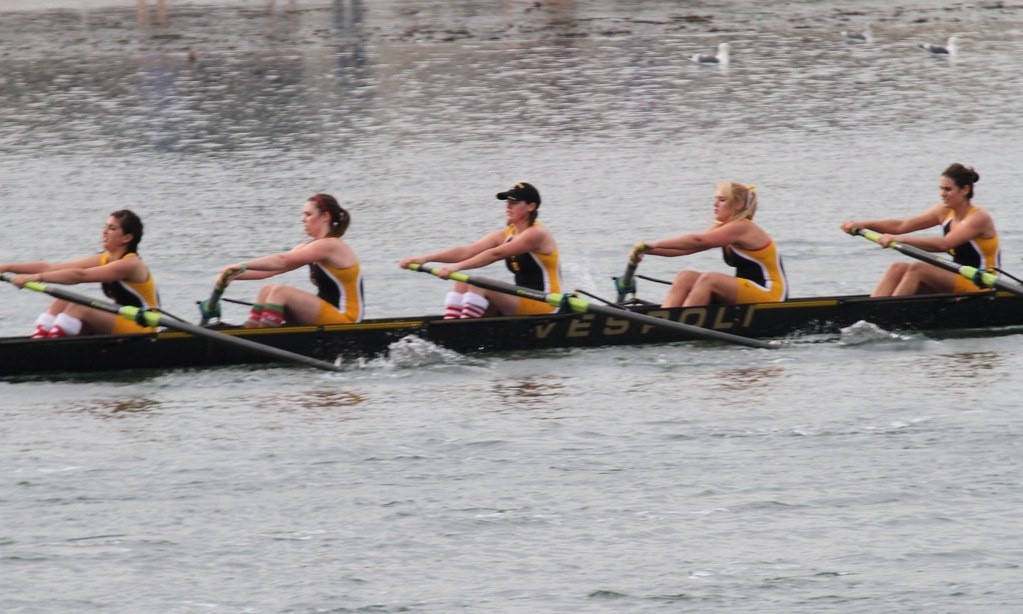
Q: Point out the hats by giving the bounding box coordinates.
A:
[496,182,541,207]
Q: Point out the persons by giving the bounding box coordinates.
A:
[843,163,1001,298]
[398,182,565,319]
[0,209,162,338]
[629,182,789,308]
[213,194,365,329]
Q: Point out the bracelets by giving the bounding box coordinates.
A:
[240,262,247,272]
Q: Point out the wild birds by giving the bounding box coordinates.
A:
[840,25,873,41]
[916,35,963,58]
[685,42,738,68]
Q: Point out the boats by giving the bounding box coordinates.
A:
[0,290,1023,377]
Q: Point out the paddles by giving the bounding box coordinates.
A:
[406,263,782,349]
[198,269,233,326]
[839,222,1023,298]
[0,273,343,370]
[615,243,645,303]
